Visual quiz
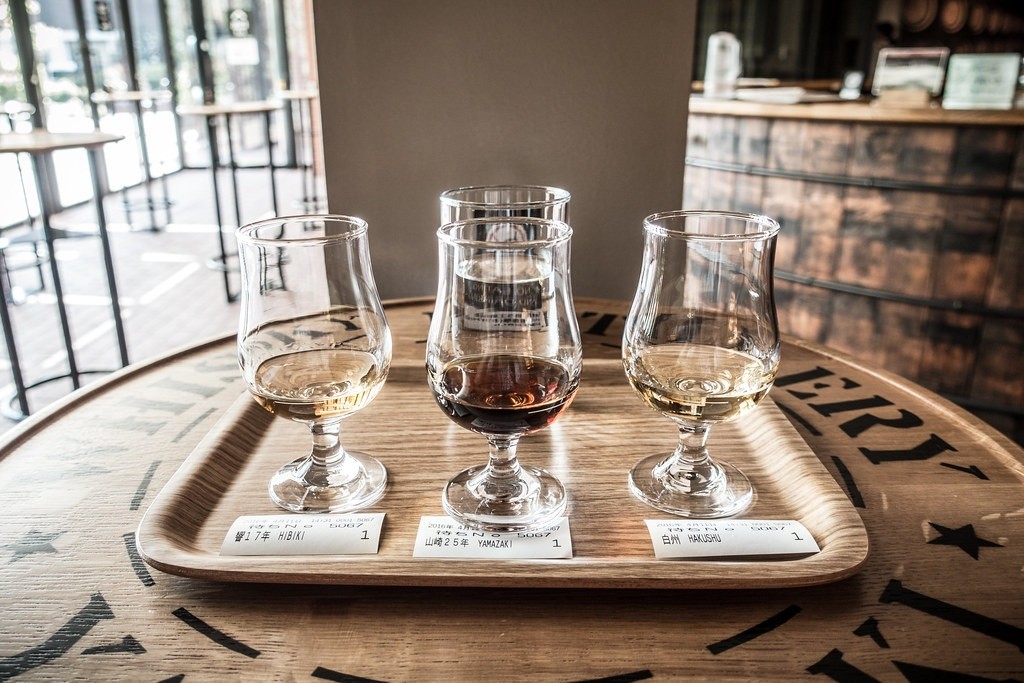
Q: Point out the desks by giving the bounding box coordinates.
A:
[175,102,288,300]
[0,295,1024,683]
[277,89,327,211]
[89,91,173,230]
[0,130,133,427]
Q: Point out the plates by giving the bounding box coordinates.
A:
[940,0,969,33]
[988,11,1004,35]
[901,0,937,32]
[970,4,988,35]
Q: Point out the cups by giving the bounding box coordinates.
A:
[704,32,739,98]
[439,185,571,373]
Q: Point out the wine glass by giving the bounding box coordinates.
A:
[236,213,393,515]
[425,219,589,537]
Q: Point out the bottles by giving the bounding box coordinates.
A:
[623,209,782,519]
